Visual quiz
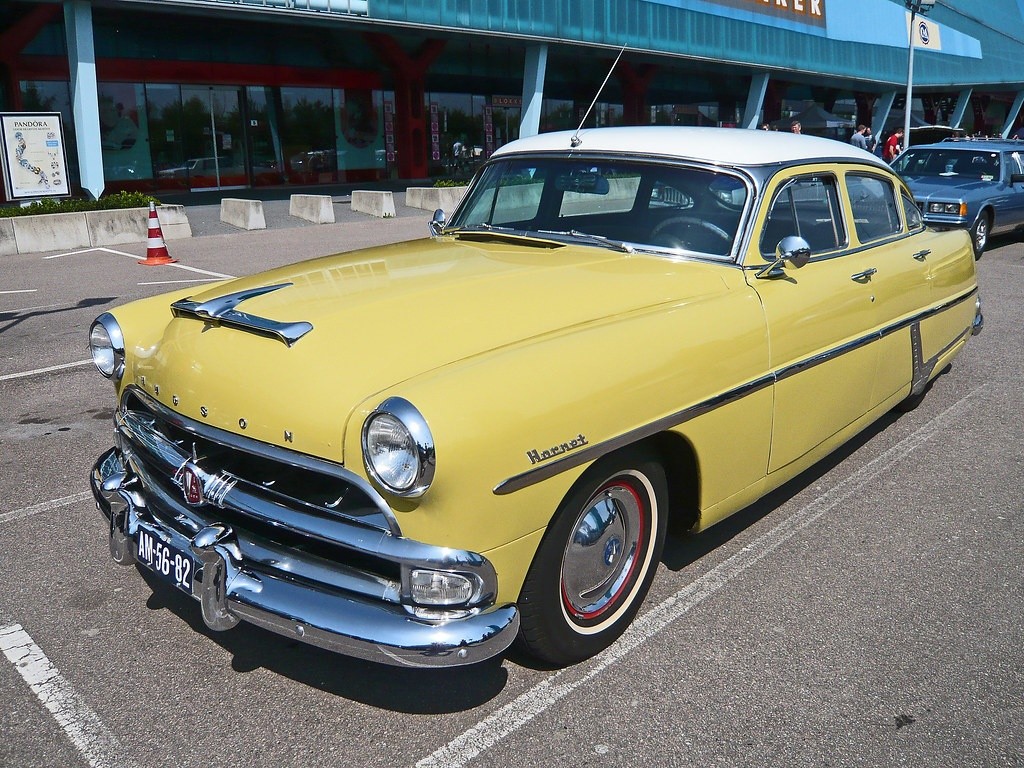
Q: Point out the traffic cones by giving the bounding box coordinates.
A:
[137,201,178,266]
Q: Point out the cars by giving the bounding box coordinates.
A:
[83,121,984,675]
[894,134,1024,259]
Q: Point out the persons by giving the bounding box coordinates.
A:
[864,128,876,153]
[952,131,961,142]
[447,137,466,176]
[761,123,770,131]
[964,132,1018,141]
[884,128,904,164]
[851,125,868,150]
[791,120,801,134]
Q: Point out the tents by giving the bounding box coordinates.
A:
[771,103,856,136]
[888,113,932,127]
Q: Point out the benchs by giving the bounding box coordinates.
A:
[675,211,819,249]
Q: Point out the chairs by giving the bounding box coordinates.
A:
[810,220,837,252]
